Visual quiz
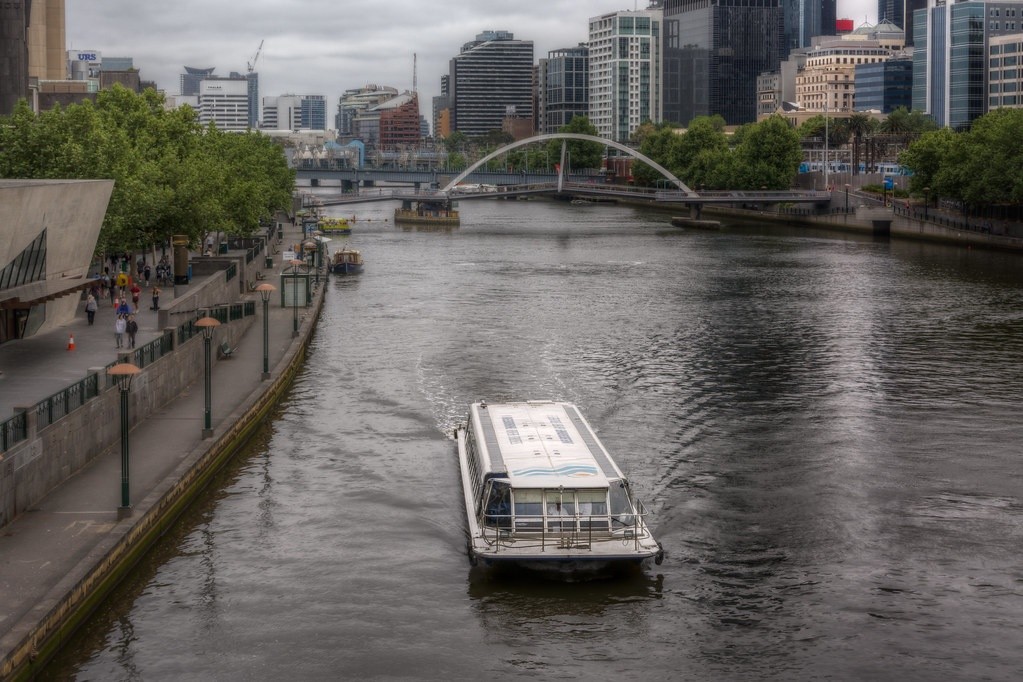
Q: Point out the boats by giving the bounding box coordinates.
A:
[454,400,664,582]
[320,224,351,234]
[334,241,366,274]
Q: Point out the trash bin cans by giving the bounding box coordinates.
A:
[266,257,273,269]
[279,224,282,230]
[220,242,228,254]
[278,232,283,240]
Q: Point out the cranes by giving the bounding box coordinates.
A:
[247,39,264,128]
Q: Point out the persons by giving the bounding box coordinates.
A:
[87,234,214,350]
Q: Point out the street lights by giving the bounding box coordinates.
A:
[311,196,315,212]
[255,283,277,372]
[923,188,930,214]
[108,363,141,506]
[289,260,304,331]
[883,181,888,205]
[194,318,221,429]
[845,184,851,212]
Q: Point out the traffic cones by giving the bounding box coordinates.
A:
[114,297,119,308]
[66,334,76,350]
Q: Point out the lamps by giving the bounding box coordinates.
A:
[623,530,634,540]
[499,531,510,539]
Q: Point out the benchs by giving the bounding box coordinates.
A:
[267,224,283,258]
[258,271,266,280]
[250,281,259,291]
[221,341,240,359]
